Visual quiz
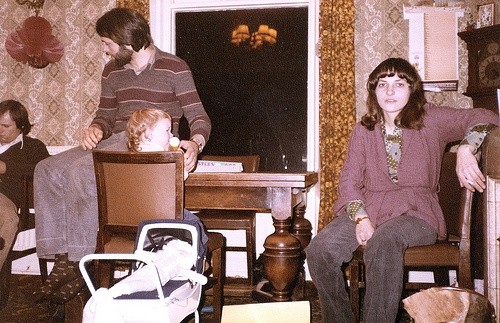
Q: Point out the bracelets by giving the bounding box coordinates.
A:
[191,138,202,154]
[355,217,369,224]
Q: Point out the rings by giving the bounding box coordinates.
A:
[362,240,366,242]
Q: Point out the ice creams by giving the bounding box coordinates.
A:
[169,137,180,152]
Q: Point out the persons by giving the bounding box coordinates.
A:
[126,108,209,274]
[306,58,500,323]
[33,8,212,305]
[0,99,51,271]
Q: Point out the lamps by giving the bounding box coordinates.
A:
[230,22,278,49]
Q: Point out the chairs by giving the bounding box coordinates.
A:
[182,152,260,296]
[0,166,48,308]
[348,149,476,323]
[91,151,227,323]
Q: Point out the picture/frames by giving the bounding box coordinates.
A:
[477,3,495,28]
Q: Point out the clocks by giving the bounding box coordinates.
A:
[457,24,500,91]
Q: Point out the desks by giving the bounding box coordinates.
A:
[114,172,318,303]
